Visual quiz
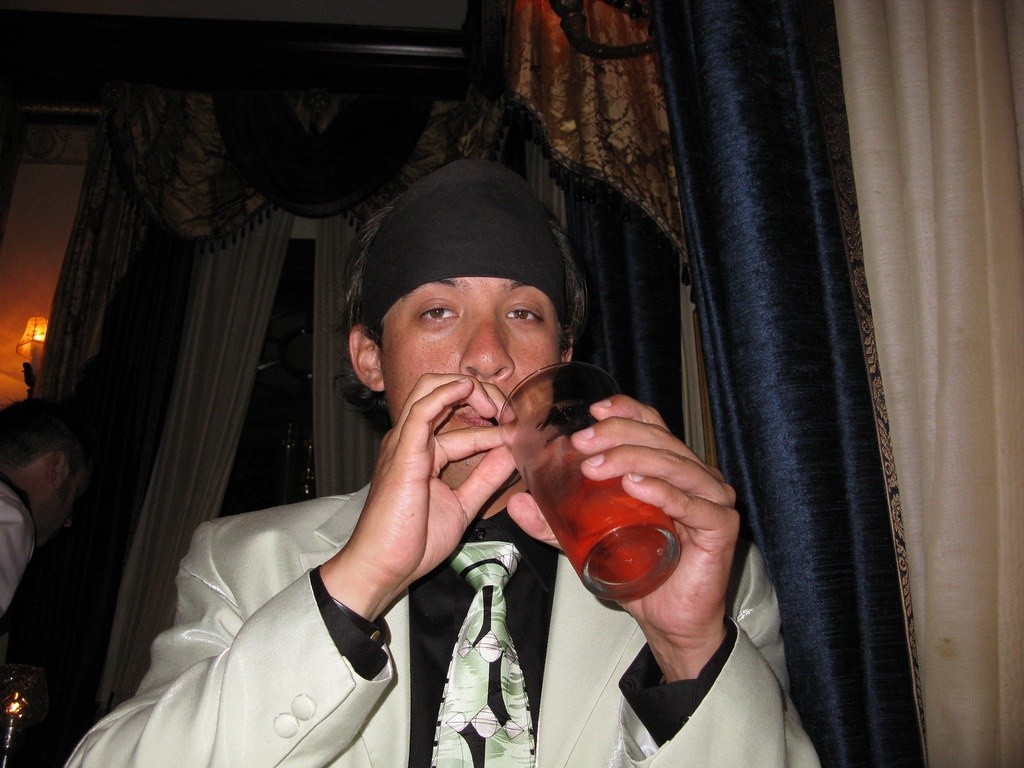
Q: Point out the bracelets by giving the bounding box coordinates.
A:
[331,597,388,646]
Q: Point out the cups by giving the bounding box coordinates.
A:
[501,362,682,601]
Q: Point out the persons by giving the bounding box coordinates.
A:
[0,396,94,620]
[61,158,822,768]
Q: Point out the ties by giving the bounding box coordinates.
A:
[429,540,536,768]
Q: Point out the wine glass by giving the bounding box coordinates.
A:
[0,663,49,768]
